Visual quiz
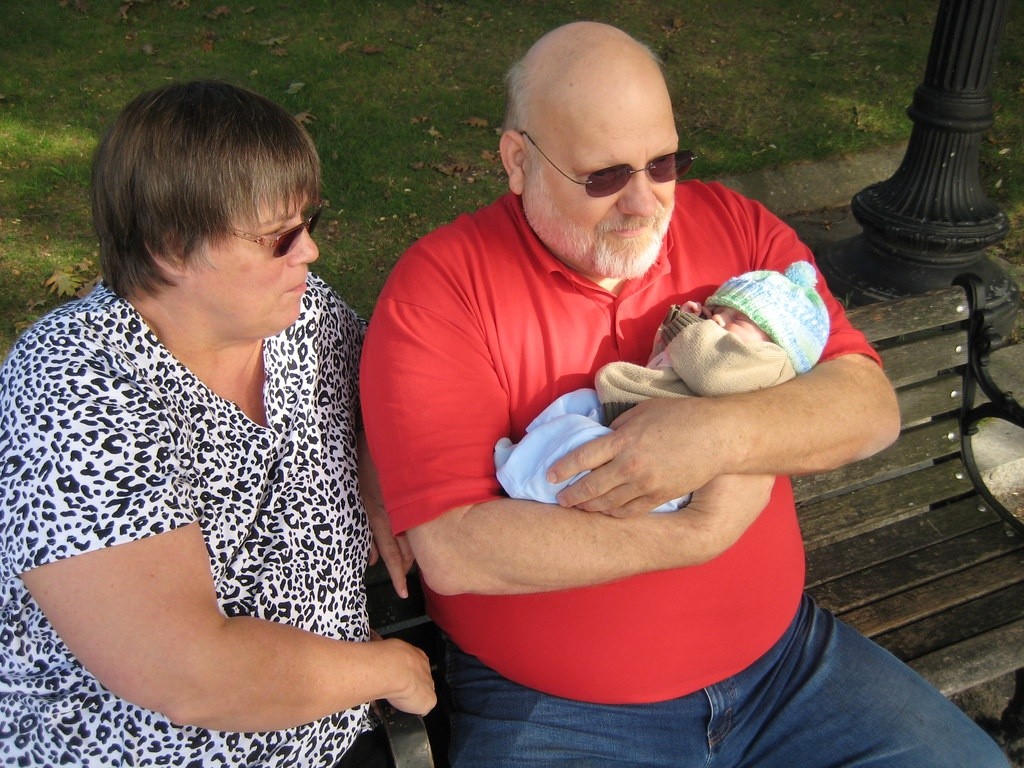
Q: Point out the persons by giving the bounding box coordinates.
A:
[358,16,1022,768]
[0,71,437,768]
[492,260,828,509]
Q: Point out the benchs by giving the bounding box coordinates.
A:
[346,284,1024,768]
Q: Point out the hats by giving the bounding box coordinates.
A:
[705,260,830,375]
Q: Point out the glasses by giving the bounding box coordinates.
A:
[177,198,327,259]
[519,129,698,201]
